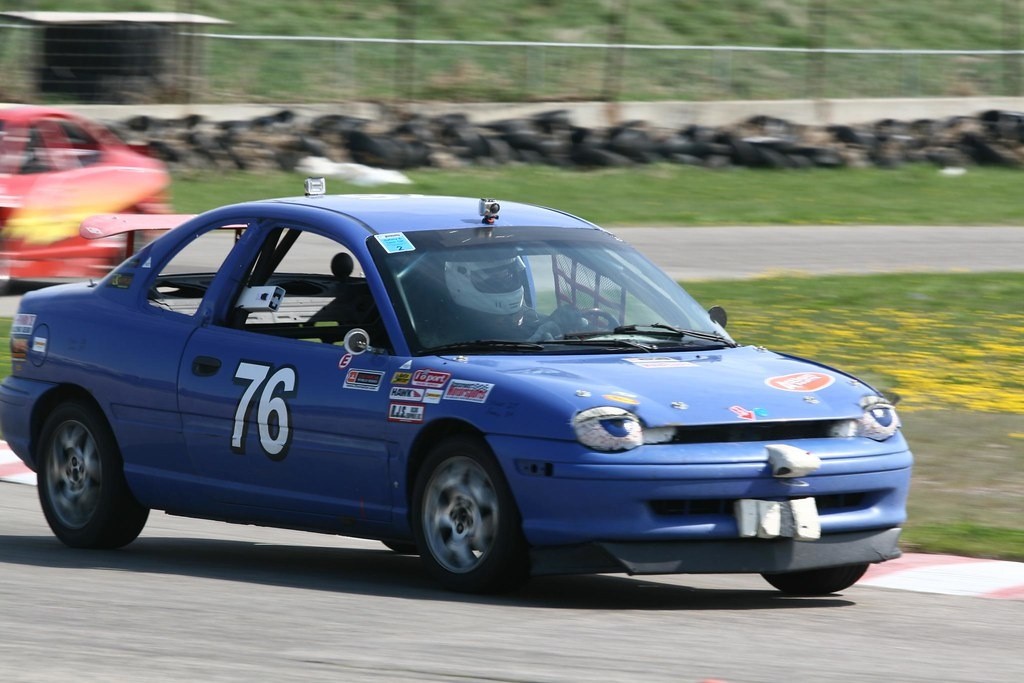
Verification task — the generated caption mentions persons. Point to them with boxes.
[444,245,588,343]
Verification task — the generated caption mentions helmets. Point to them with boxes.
[444,256,526,315]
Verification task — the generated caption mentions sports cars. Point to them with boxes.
[0,110,179,288]
[0,195,914,595]
